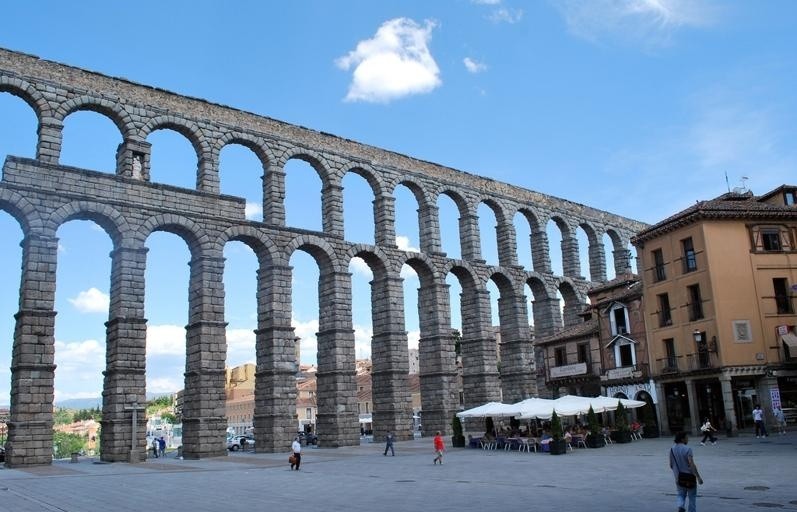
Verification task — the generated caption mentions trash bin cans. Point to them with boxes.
[71,453,78,463]
[301,437,307,447]
[178,446,183,457]
[244,441,249,451]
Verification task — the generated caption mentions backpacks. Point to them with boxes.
[701,425,709,432]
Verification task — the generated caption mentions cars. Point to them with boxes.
[297,430,317,447]
[227,435,255,451]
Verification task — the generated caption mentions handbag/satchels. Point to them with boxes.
[678,471,696,491]
[288,455,296,464]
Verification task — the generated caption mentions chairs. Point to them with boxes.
[479,425,644,453]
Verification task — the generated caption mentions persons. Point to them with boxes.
[752,404,765,439]
[774,406,787,434]
[700,417,717,445]
[382,430,395,456]
[669,431,703,512]
[484,420,641,452]
[290,436,300,471]
[433,431,445,465]
[152,436,165,458]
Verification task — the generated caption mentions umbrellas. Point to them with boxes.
[456,394,647,437]
